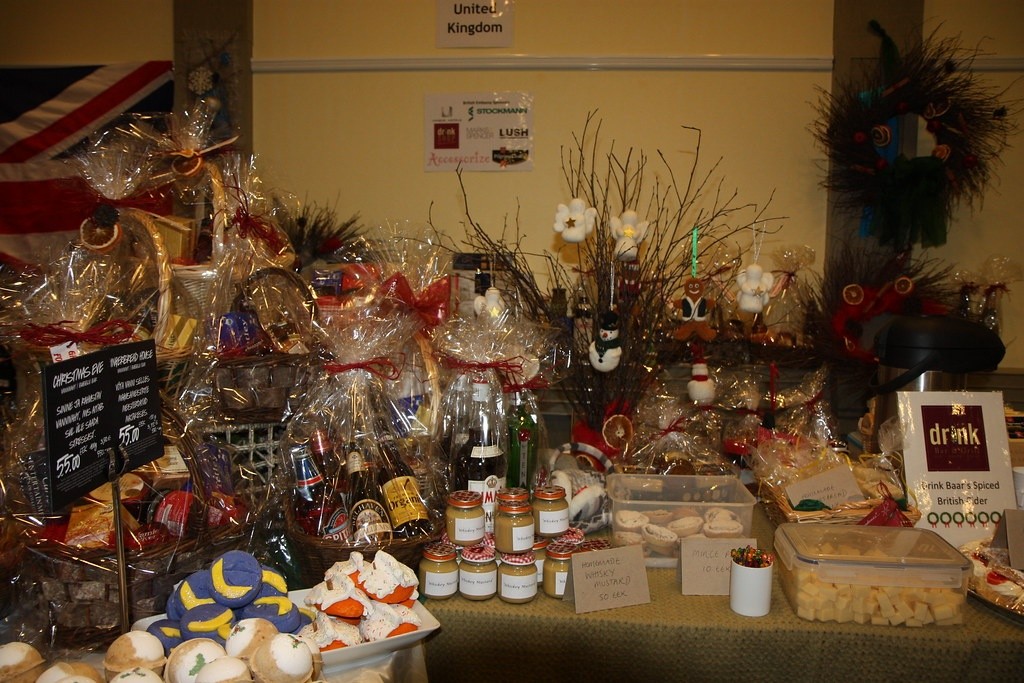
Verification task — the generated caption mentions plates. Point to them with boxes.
[288,589,441,673]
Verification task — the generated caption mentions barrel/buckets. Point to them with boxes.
[877,363,951,445]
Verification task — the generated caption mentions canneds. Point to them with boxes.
[420,485,615,603]
[75,475,206,551]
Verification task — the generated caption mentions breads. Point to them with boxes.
[614,507,746,559]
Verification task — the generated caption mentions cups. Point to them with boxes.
[730,558,773,616]
[1013,467,1024,509]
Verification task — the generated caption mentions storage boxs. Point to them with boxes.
[606,473,757,568]
[773,523,974,628]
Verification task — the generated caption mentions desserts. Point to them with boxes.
[0,551,418,683]
[958,546,1024,615]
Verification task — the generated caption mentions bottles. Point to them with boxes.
[578,280,593,310]
[294,389,534,543]
